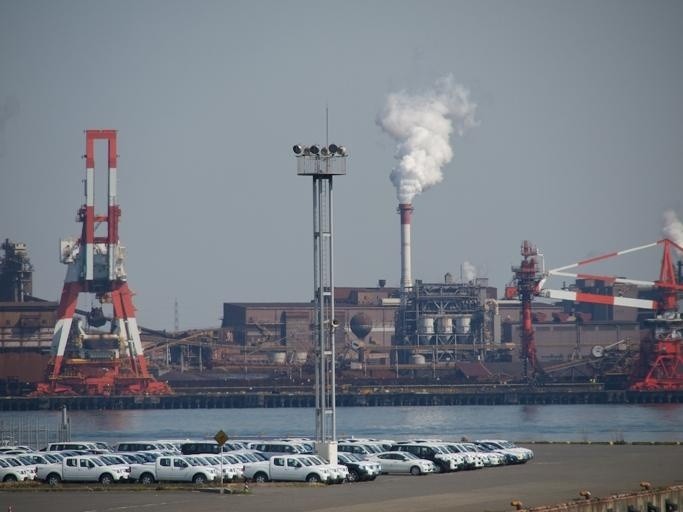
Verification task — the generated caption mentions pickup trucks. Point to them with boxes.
[0,435,535,487]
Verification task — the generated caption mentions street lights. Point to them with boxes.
[291,104,348,469]
